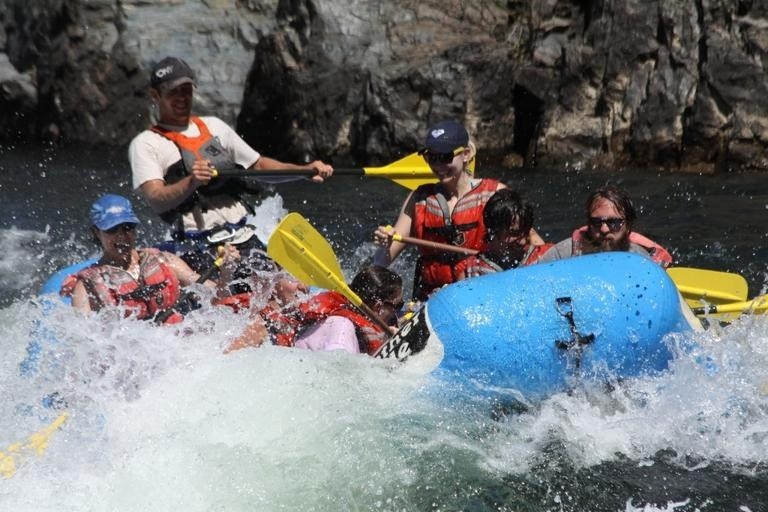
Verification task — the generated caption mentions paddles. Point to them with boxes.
[267,212,394,338]
[212,146,476,192]
[0,246,236,481]
[384,226,768,324]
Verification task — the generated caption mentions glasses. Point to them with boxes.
[97,223,137,233]
[589,218,626,232]
[383,300,405,312]
[425,147,464,164]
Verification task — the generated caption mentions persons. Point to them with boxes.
[58,193,218,327]
[453,188,556,281]
[373,120,545,302]
[290,264,403,354]
[226,272,311,353]
[128,56,334,296]
[529,186,673,273]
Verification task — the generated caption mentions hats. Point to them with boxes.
[418,123,468,155]
[89,194,140,230]
[151,56,197,94]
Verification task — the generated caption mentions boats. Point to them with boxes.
[21,251,720,419]
